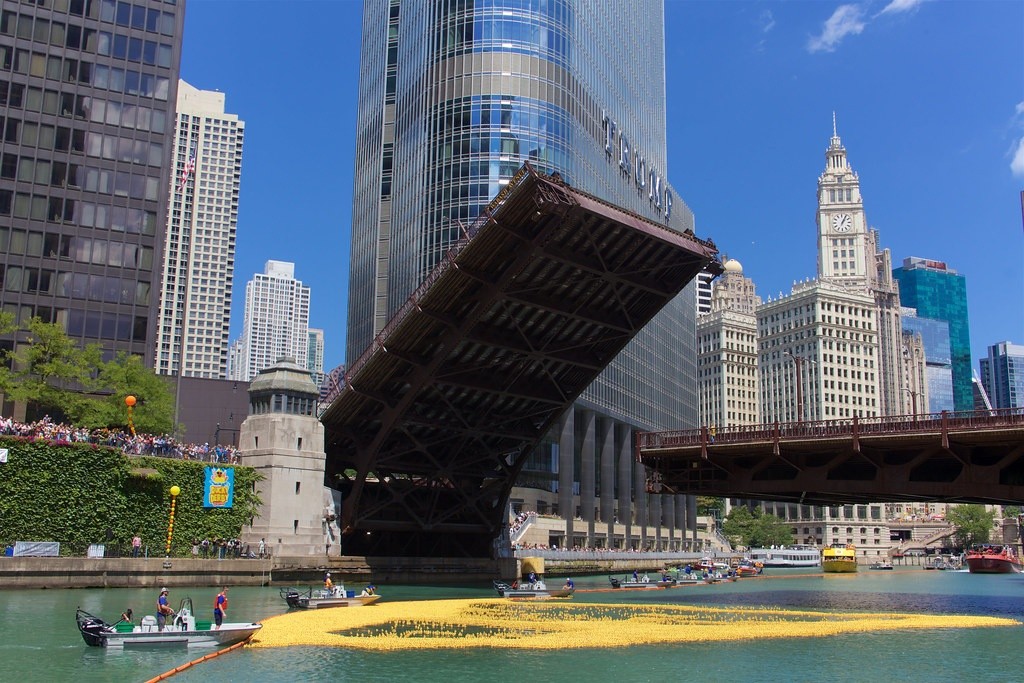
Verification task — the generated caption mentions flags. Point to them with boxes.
[176,149,195,191]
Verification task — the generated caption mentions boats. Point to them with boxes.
[280,582,383,609]
[869,559,893,570]
[923,553,962,570]
[608,571,674,589]
[493,579,576,598]
[745,544,821,567]
[965,543,1024,573]
[673,556,763,586]
[75,595,263,649]
[820,543,858,573]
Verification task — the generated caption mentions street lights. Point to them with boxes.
[783,352,817,426]
[901,387,925,422]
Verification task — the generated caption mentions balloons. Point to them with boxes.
[125,396,136,406]
[165,485,181,553]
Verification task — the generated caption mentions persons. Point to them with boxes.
[361,585,377,596]
[325,572,335,594]
[632,570,638,580]
[192,538,200,559]
[121,608,133,624]
[563,577,574,591]
[969,545,1014,560]
[889,513,945,524]
[212,536,218,558]
[258,538,266,560]
[528,571,538,584]
[202,538,210,559]
[762,544,798,551]
[156,587,173,631]
[219,537,256,559]
[512,580,519,589]
[132,535,142,558]
[710,423,716,444]
[213,586,228,630]
[510,511,690,553]
[661,560,763,581]
[0,414,239,465]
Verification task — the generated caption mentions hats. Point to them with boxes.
[161,587,169,592]
